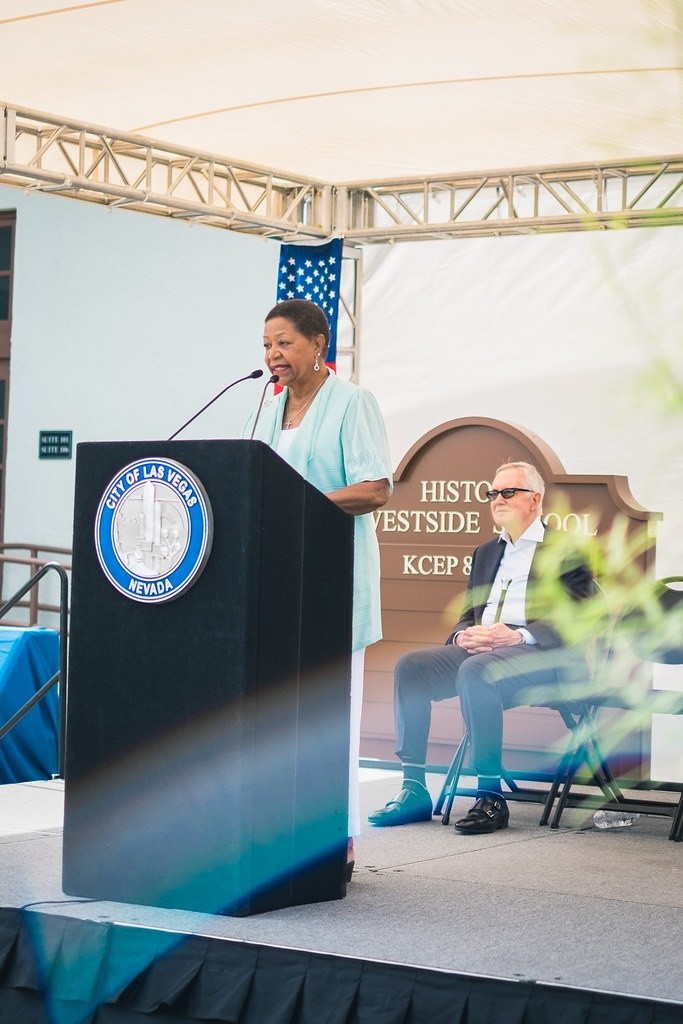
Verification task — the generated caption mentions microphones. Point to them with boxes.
[250,375,280,439]
[168,370,264,440]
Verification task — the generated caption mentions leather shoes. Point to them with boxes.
[368,790,433,826]
[454,798,509,834]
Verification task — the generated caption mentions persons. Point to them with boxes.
[238,300,394,883]
[367,461,593,834]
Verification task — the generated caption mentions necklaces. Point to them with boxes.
[284,374,329,430]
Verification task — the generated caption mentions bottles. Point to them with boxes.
[593,810,641,829]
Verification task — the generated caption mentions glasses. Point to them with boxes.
[484,488,534,501]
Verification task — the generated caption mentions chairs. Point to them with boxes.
[432,582,622,830]
[550,575,683,840]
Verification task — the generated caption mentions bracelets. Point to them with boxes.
[519,636,526,644]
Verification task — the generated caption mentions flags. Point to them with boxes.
[273,233,345,396]
